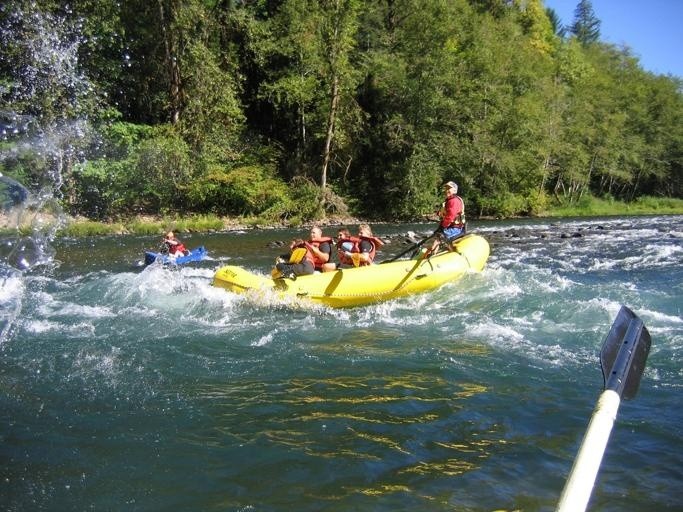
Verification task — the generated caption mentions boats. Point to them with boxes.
[214,234,490,307]
[145,246,207,265]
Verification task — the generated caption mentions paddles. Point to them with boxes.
[289,246,306,264]
[379,233,437,264]
[553,305,652,512]
[339,250,360,268]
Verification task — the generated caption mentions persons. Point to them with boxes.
[277,239,315,275]
[335,229,350,263]
[340,224,384,268]
[306,226,333,271]
[422,181,466,259]
[166,231,189,258]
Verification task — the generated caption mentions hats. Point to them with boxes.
[443,181,458,193]
